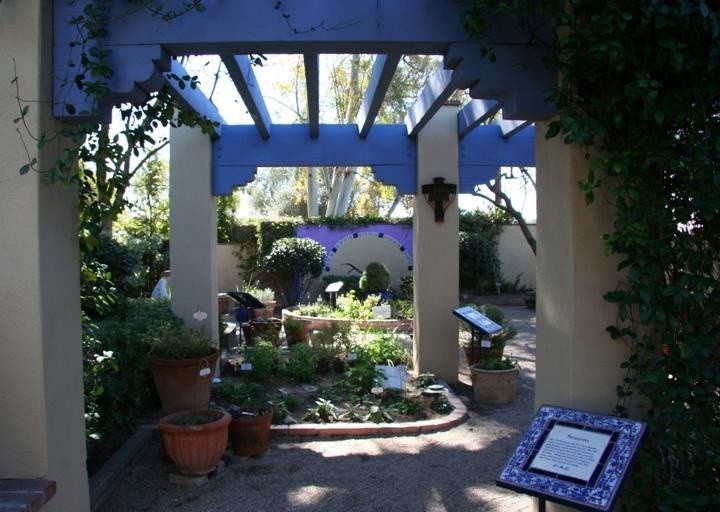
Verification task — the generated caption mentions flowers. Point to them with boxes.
[135,301,222,356]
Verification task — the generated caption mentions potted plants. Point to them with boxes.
[223,271,260,321]
[213,380,275,459]
[466,317,522,407]
[158,407,233,479]
[461,301,506,369]
[239,284,279,319]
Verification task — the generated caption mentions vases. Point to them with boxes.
[146,347,219,418]
[284,318,310,347]
[241,317,282,347]
